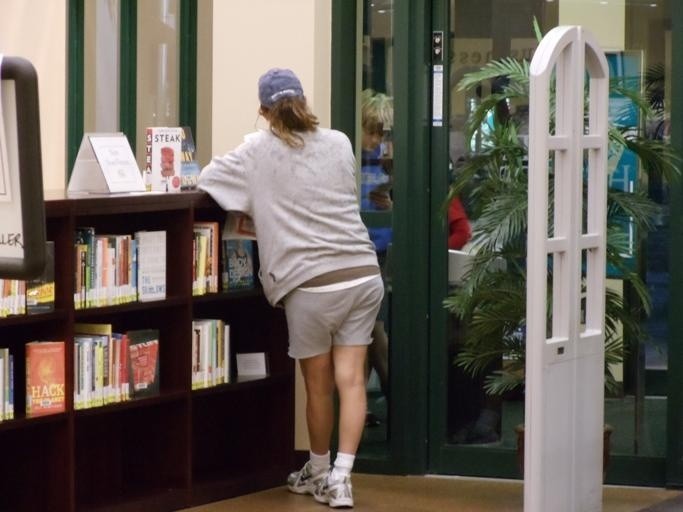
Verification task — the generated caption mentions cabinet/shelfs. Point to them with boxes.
[0,189,297,512]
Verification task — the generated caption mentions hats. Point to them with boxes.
[258,67,304,110]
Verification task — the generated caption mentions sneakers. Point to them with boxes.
[287,459,354,508]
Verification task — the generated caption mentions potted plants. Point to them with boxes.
[436,11,682,487]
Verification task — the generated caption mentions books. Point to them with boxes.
[1,241,55,318]
[191,319,230,390]
[193,222,253,295]
[74,323,159,410]
[73,227,167,309]
[0,341,64,422]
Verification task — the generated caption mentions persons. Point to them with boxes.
[353,85,392,428]
[195,65,383,508]
